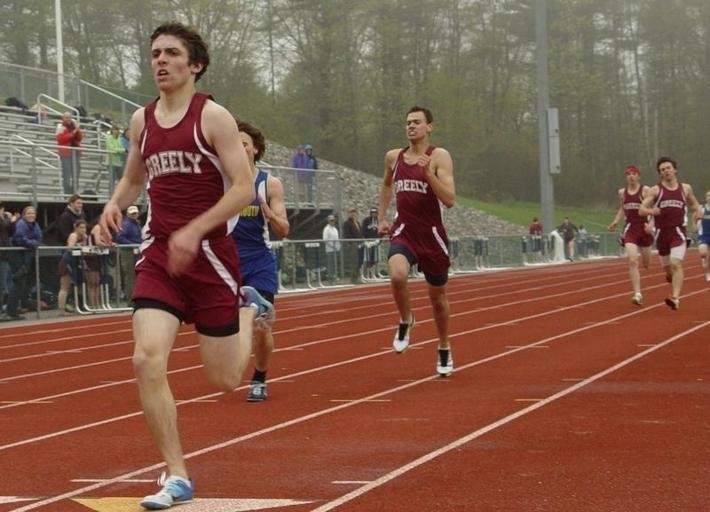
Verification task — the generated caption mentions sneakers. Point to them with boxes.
[393,316,415,353]
[139,476,196,507]
[246,376,268,402]
[239,287,273,316]
[630,291,642,307]
[665,295,681,311]
[437,342,456,378]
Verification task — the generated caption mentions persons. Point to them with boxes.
[60,196,85,244]
[226,121,289,401]
[558,217,578,262]
[58,221,86,317]
[87,219,113,312]
[695,191,707,281]
[103,123,137,198]
[12,206,41,314]
[374,105,456,376]
[57,112,82,195]
[115,205,142,302]
[638,158,703,309]
[529,218,541,234]
[363,207,382,281]
[293,141,319,201]
[609,165,654,306]
[325,216,341,281]
[1,205,26,320]
[100,23,276,507]
[341,207,366,282]
[578,226,588,253]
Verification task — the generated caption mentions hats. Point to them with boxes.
[127,206,140,215]
[305,144,312,150]
